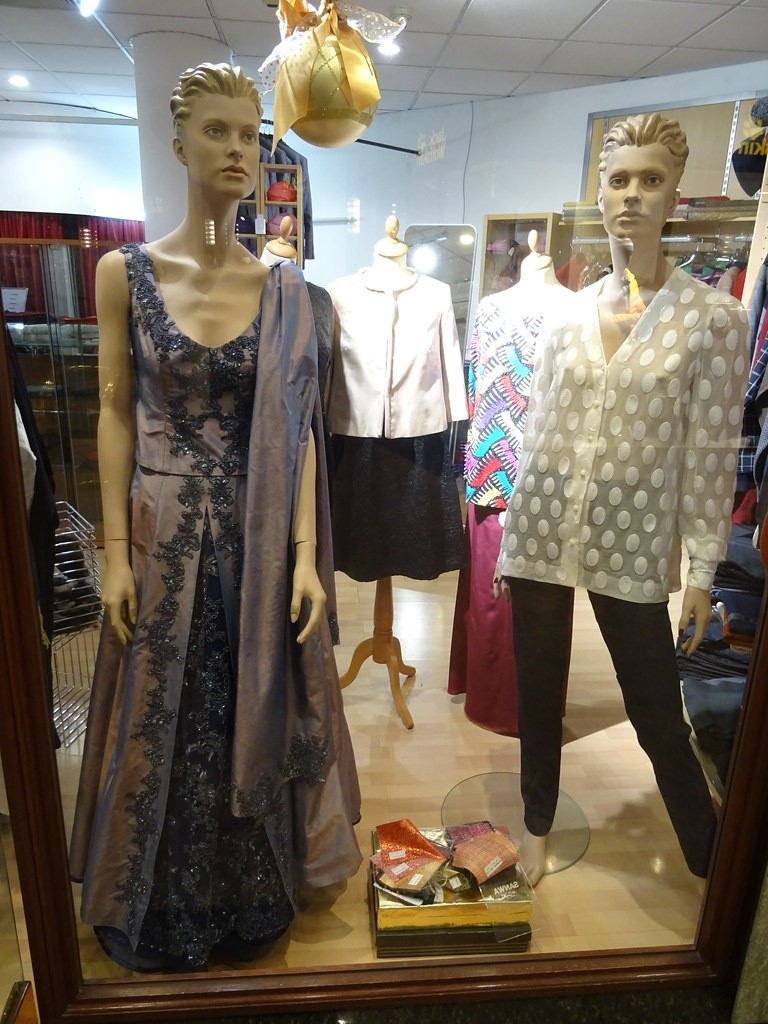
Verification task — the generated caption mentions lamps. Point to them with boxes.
[258,2,407,158]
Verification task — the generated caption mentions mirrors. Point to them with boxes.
[402,225,479,468]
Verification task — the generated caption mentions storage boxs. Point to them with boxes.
[365,822,537,958]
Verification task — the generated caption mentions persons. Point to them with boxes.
[0,313,63,748]
[251,236,580,739]
[95,63,327,972]
[497,113,751,892]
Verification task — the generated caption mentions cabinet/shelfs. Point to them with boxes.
[467,213,561,366]
